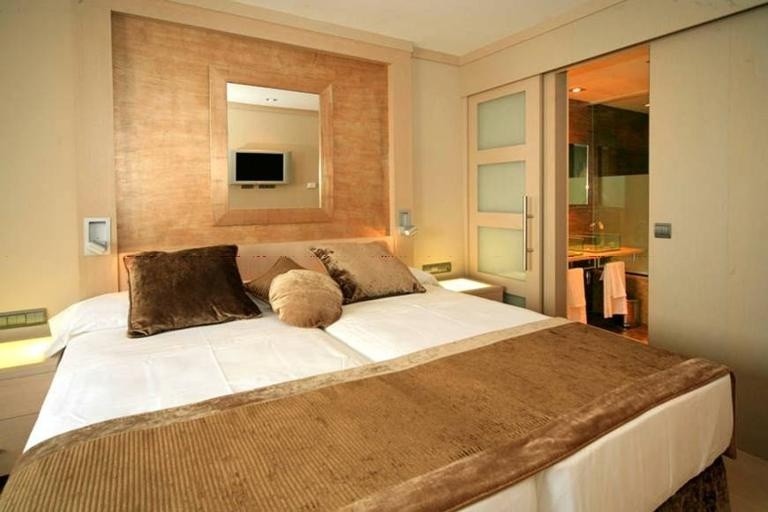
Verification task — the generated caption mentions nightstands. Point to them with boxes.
[0,323,64,476]
[438,277,505,302]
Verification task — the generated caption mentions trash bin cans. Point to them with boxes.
[624,300,641,330]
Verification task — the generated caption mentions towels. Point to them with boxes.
[568,142,590,206]
[603,261,628,318]
[567,268,587,324]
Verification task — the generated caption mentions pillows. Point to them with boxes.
[235,244,330,283]
[306,243,426,303]
[269,269,341,329]
[46,291,131,355]
[122,240,261,339]
[244,258,305,302]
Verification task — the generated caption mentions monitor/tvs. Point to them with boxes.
[226,148,291,186]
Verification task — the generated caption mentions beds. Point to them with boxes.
[0,284,737,512]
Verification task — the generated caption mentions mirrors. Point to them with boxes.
[208,64,335,225]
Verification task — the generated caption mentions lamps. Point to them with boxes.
[84,217,111,257]
[398,208,419,240]
